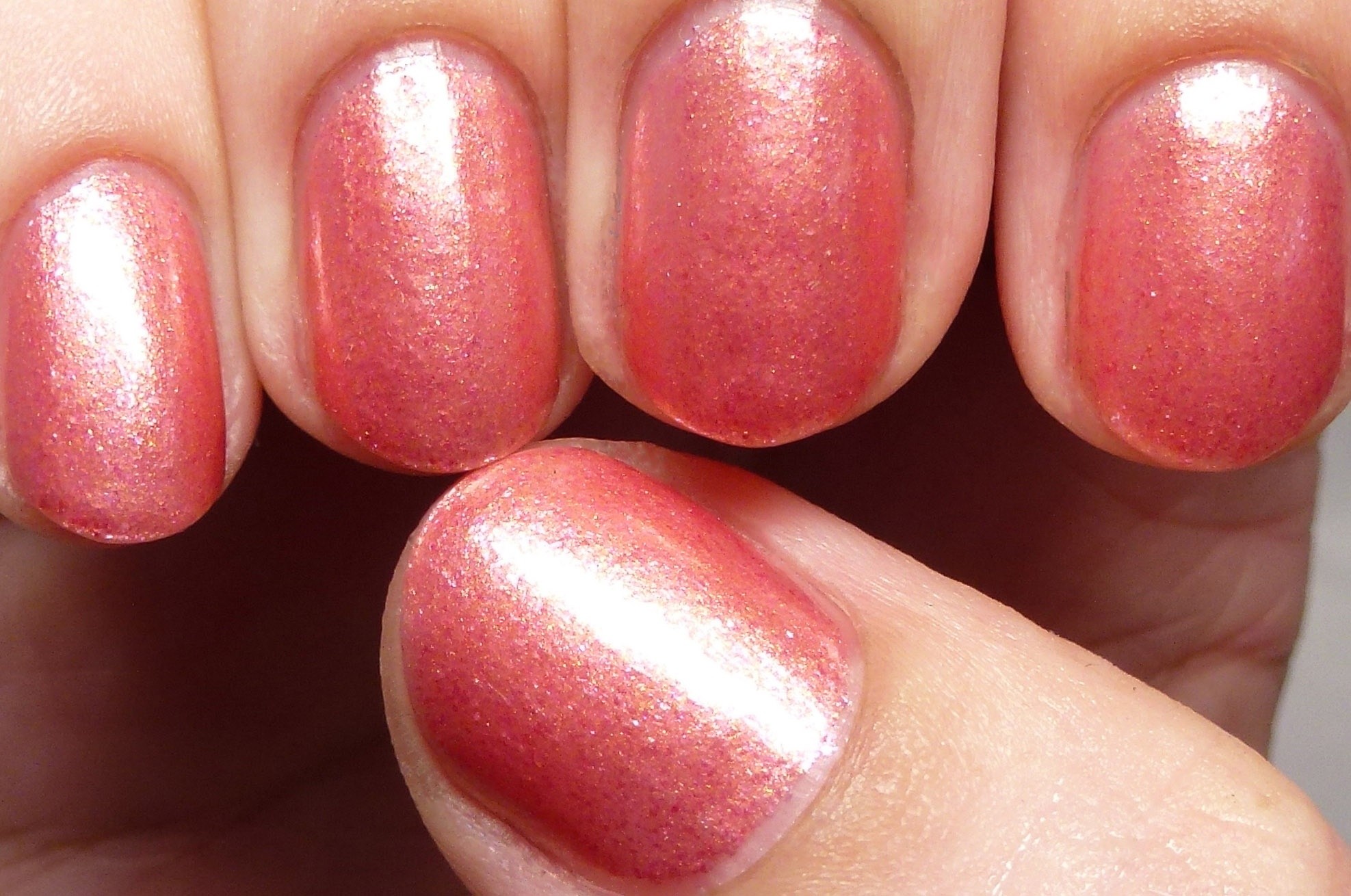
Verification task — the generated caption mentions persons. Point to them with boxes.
[1,0,1351,895]
